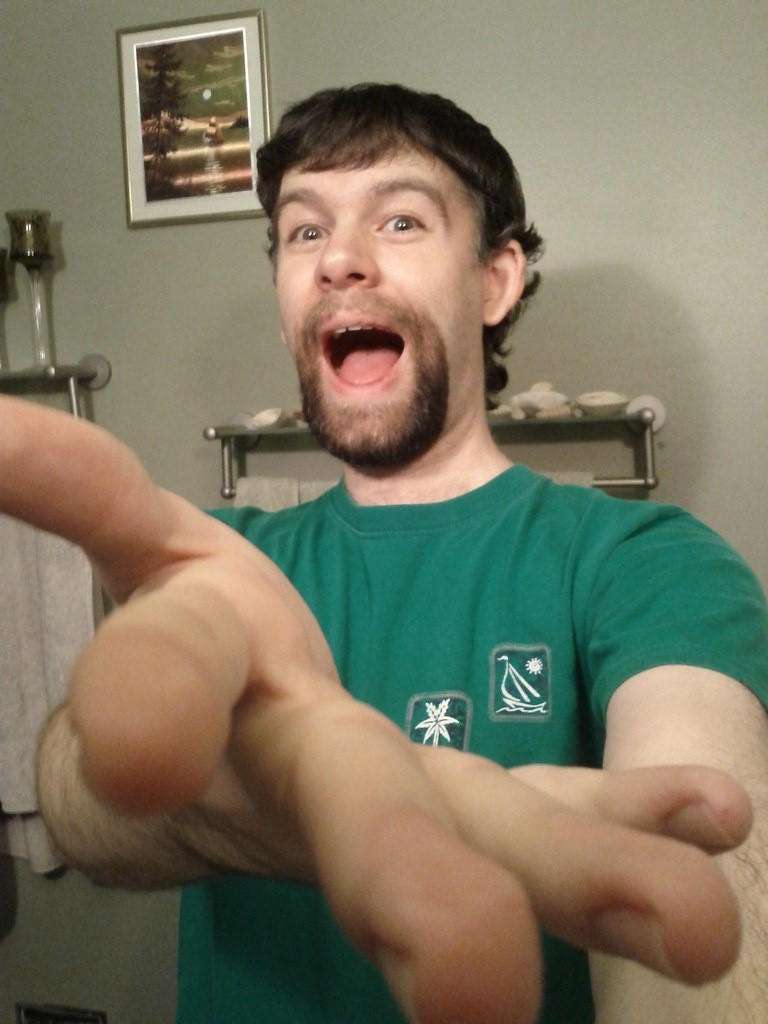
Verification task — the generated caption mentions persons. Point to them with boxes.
[0,81,768,1024]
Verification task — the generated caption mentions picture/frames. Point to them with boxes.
[114,7,275,231]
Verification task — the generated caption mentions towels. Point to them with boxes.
[0,495,148,877]
